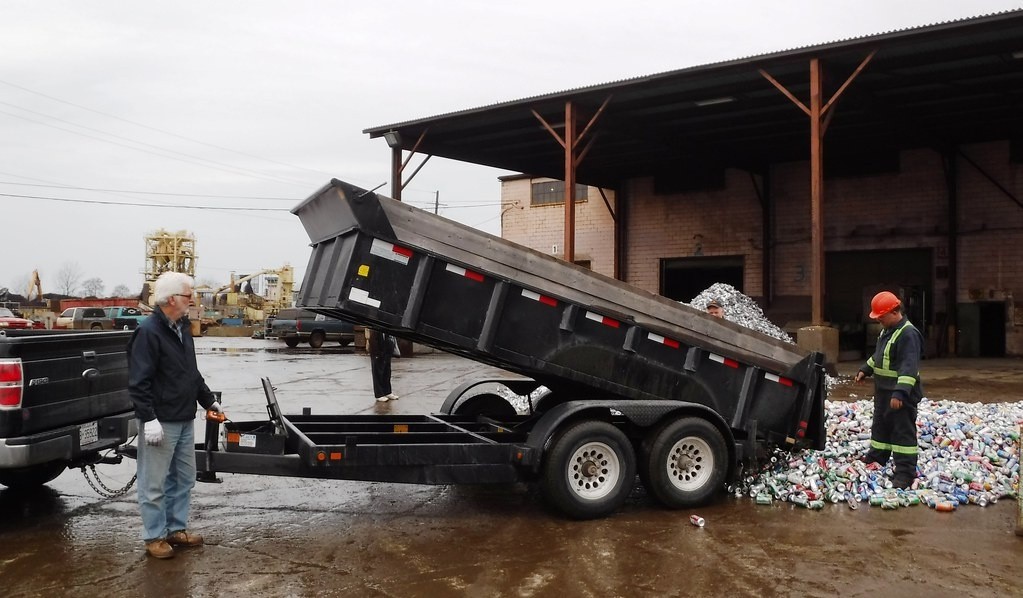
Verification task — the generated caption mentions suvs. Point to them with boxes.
[263,306,374,348]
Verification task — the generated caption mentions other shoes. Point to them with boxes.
[386,394,399,400]
[376,396,389,401]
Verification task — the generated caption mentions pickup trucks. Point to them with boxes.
[0,329,137,487]
[82,306,154,331]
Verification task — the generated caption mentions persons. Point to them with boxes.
[125,272,224,559]
[369,323,399,402]
[706,300,726,321]
[851,290,924,490]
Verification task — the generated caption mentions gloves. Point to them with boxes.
[208,402,224,416]
[145,418,164,446]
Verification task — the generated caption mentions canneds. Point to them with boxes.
[690,515,705,526]
[724,399,1023,511]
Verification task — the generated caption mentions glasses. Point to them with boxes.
[172,293,191,299]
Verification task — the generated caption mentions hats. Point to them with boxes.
[706,299,723,308]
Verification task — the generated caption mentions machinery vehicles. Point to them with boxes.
[27,266,52,309]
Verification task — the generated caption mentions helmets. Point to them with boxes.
[869,291,901,319]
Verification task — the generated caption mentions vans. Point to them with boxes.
[56,306,106,330]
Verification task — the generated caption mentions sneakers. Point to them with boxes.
[167,531,204,546]
[145,540,174,558]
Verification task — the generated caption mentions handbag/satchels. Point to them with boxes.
[390,336,400,356]
